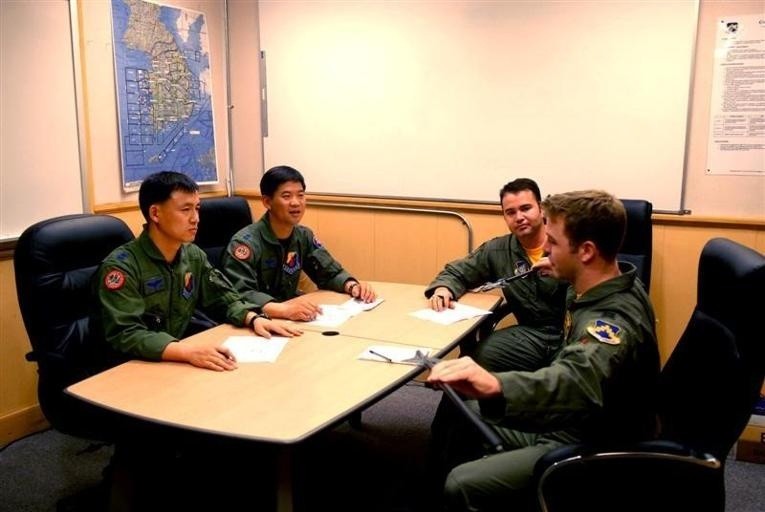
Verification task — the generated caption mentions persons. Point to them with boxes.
[90,170,303,374]
[426,189,663,510]
[219,166,377,322]
[424,177,572,445]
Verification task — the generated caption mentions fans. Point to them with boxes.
[258,0,702,215]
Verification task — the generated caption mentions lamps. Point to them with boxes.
[193,197,251,324]
[535,239,765,512]
[620,199,652,295]
[15,213,211,445]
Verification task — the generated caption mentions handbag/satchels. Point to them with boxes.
[348,283,360,294]
[249,313,270,329]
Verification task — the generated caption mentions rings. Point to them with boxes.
[437,294,458,302]
[220,352,235,362]
[369,350,392,363]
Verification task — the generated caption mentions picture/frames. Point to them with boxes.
[65,280,502,512]
